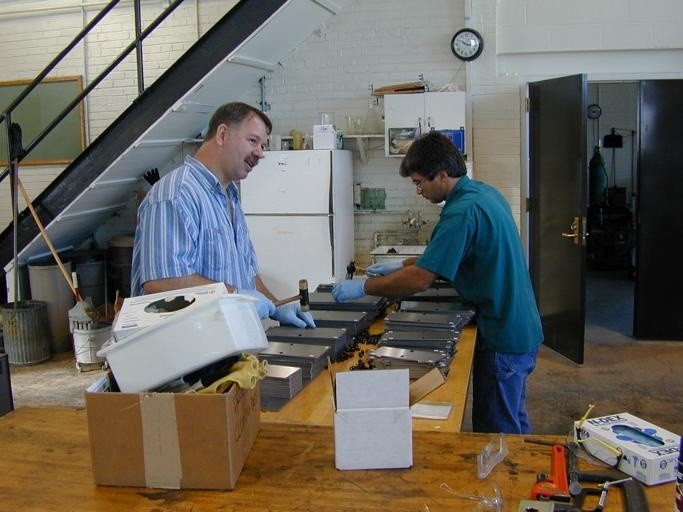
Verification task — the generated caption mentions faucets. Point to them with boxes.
[406,209,423,232]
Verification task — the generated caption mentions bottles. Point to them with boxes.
[336,128,343,150]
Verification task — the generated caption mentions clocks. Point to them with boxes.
[450,28,484,61]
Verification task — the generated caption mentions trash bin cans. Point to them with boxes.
[586,234,613,271]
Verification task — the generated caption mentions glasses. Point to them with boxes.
[411,173,428,191]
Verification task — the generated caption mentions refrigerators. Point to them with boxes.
[239,149,355,301]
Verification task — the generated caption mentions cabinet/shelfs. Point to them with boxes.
[384,91,466,158]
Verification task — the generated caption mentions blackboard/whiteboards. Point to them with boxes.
[1,75,86,165]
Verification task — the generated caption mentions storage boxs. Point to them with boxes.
[96,293,271,394]
[84,354,260,491]
[326,355,446,470]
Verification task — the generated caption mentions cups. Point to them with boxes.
[292,131,303,150]
[268,134,282,151]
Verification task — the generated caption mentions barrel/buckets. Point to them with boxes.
[73,322,112,373]
[0,301,52,365]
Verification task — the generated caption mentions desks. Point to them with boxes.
[263,303,477,432]
[0,405,676,512]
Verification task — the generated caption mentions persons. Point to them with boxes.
[129,101,319,329]
[330,129,545,435]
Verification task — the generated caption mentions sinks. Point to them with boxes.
[370,245,426,257]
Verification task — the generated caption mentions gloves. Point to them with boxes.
[331,278,370,305]
[237,289,277,320]
[270,299,317,329]
[365,260,405,278]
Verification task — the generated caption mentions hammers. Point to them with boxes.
[276,280,310,312]
[525,437,578,451]
[346,259,381,280]
